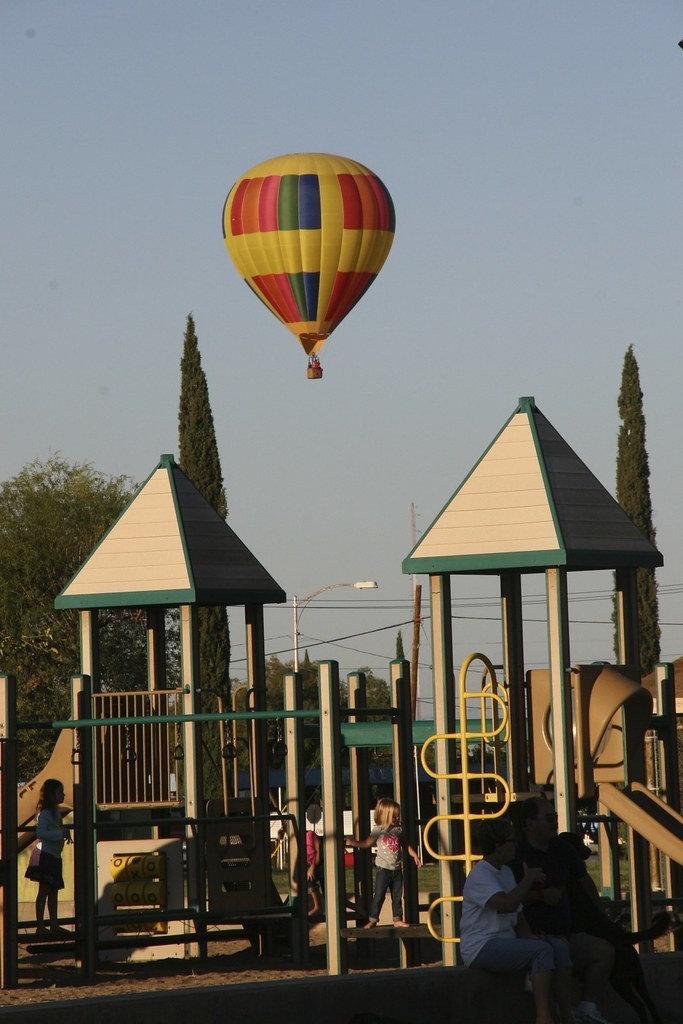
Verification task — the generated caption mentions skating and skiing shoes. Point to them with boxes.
[572,1007,607,1024]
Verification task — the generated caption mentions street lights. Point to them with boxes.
[293,581,379,673]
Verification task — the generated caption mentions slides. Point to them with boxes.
[594,780,683,866]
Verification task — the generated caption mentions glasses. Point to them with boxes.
[532,814,558,823]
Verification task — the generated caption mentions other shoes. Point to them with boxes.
[35,926,70,933]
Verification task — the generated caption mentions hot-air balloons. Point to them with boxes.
[222,152,397,356]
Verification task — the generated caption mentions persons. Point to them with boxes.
[461,796,616,1024]
[305,830,325,917]
[24,779,74,935]
[345,799,423,929]
